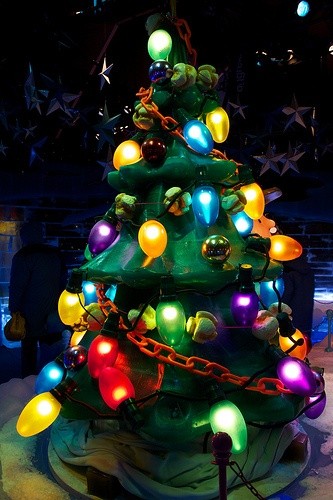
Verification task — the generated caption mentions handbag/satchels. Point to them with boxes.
[4,311,26,341]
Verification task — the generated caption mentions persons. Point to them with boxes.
[9,223,68,379]
[279,252,314,349]
